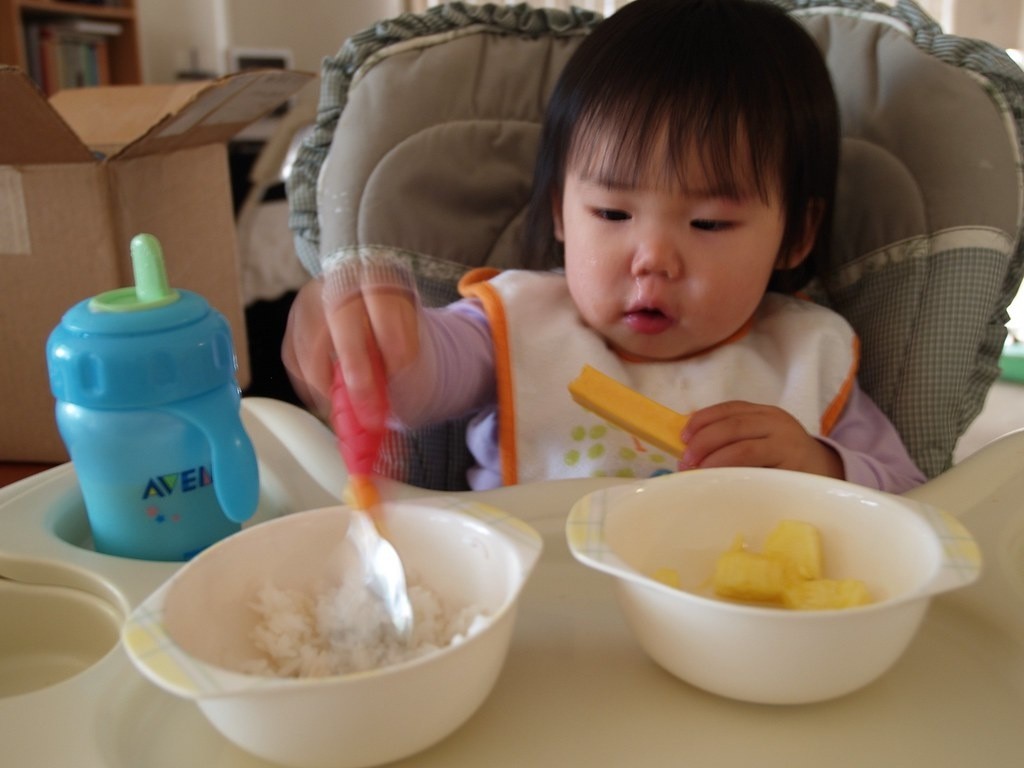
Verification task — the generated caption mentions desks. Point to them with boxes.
[0,396,1023,768]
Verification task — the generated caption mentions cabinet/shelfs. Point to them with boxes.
[0,0,142,93]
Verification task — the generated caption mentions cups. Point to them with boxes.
[0,556,130,768]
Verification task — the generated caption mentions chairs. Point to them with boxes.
[236,111,315,311]
[283,0,1024,491]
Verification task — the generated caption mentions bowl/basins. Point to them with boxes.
[563,467,983,710]
[121,492,544,768]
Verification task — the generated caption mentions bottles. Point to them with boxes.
[47,232,263,559]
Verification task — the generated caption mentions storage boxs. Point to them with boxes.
[0,65,318,464]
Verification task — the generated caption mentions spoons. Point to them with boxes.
[331,345,414,642]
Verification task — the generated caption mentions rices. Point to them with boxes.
[222,579,493,678]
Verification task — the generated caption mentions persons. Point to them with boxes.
[282,0,924,491]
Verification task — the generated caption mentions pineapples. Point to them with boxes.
[643,518,872,613]
